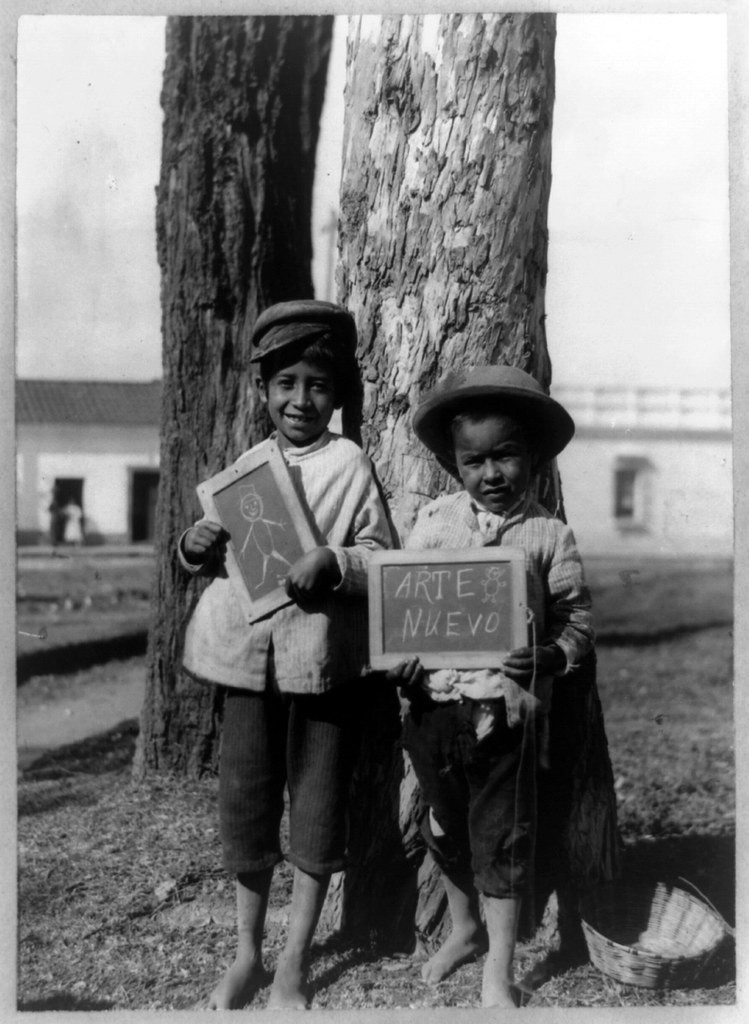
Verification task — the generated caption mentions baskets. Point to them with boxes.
[577,878,725,989]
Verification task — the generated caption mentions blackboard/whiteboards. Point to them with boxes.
[195,438,319,624]
[368,550,529,670]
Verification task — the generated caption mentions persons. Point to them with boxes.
[46,496,86,555]
[385,367,593,1009]
[178,300,396,1013]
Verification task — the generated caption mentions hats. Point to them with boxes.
[249,300,354,364]
[412,365,575,467]
